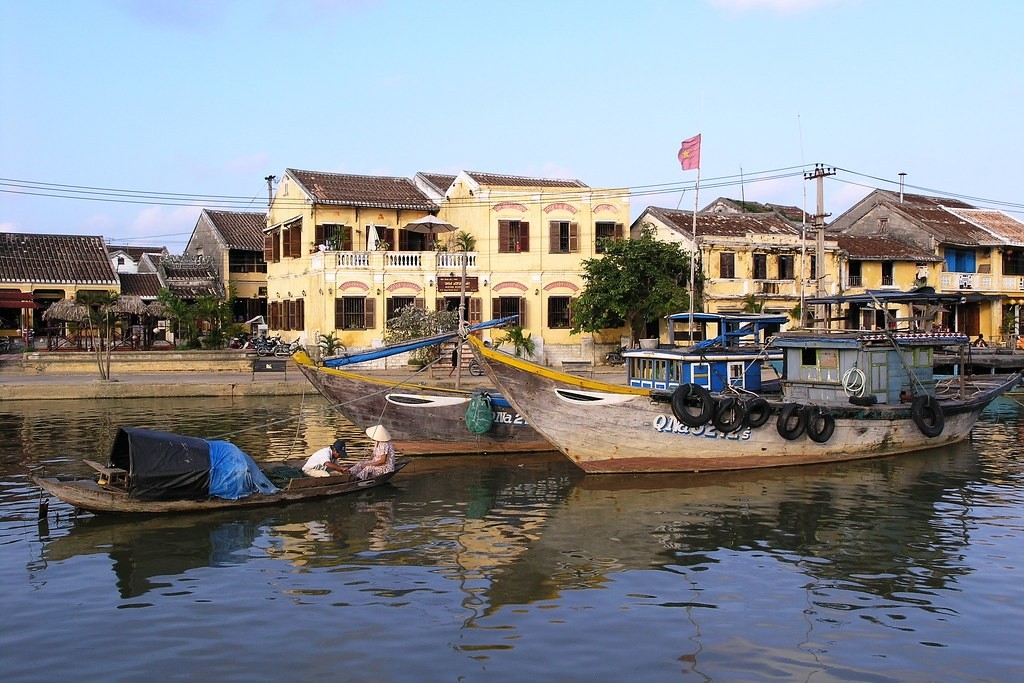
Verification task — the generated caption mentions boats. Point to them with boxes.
[291,314,562,455]
[32,426,412,512]
[465,312,1022,468]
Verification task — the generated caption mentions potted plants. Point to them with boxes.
[407,358,422,372]
[638,335,658,350]
[315,331,347,356]
[377,239,389,251]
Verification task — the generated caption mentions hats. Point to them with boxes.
[365,424,391,442]
[484,341,489,344]
[334,440,347,458]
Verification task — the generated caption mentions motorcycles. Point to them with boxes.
[0,336,20,354]
[251,333,305,358]
[469,357,484,377]
[605,345,628,367]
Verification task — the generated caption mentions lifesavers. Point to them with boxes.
[777,402,808,441]
[911,394,945,437]
[712,397,744,432]
[806,407,835,443]
[671,383,714,428]
[743,397,771,429]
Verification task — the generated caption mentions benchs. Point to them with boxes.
[85,460,128,489]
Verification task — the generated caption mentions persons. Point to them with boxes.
[448,342,463,378]
[972,334,988,348]
[302,441,349,478]
[349,425,395,480]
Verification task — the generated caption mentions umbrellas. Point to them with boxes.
[403,213,460,241]
[367,222,381,251]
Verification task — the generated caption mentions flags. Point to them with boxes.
[678,134,701,171]
